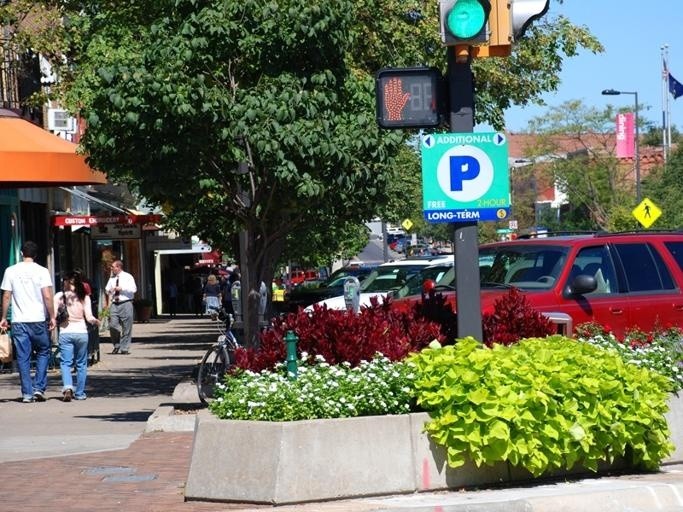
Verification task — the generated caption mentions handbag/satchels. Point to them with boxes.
[0,328,14,363]
[55,289,69,325]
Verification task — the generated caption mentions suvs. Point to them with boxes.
[378,227,682,344]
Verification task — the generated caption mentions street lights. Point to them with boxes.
[514,158,561,224]
[599,89,643,233]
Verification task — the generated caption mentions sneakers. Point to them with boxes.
[112,347,129,355]
[22,390,87,402]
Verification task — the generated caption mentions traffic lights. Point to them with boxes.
[487,0,550,47]
[375,65,441,128]
[439,0,492,49]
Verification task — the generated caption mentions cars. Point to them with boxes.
[283,235,551,348]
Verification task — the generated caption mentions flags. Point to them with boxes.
[664,59,683,99]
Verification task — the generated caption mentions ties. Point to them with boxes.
[115,277,119,302]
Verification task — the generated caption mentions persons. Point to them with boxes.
[73,268,92,298]
[104,260,138,354]
[167,270,240,321]
[0,241,55,403]
[51,272,101,402]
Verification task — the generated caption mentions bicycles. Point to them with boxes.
[196,303,268,408]
